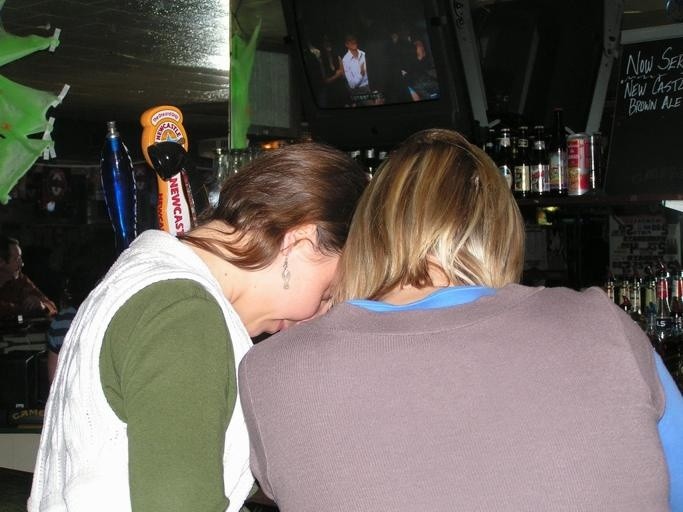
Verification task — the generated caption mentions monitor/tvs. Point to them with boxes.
[283,0,469,138]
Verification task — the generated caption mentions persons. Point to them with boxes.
[45,236,114,386]
[341,33,369,91]
[237,127,683,511]
[26,141,372,511]
[0,238,58,318]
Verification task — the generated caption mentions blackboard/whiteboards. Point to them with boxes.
[603,37,683,196]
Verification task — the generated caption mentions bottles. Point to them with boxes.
[484,128,497,160]
[514,126,530,198]
[567,132,594,195]
[549,108,566,194]
[498,129,514,196]
[670,270,683,375]
[229,145,240,178]
[607,278,614,303]
[630,276,647,332]
[530,126,549,198]
[652,272,679,380]
[365,147,374,181]
[204,146,227,207]
[644,275,656,349]
[618,280,631,306]
